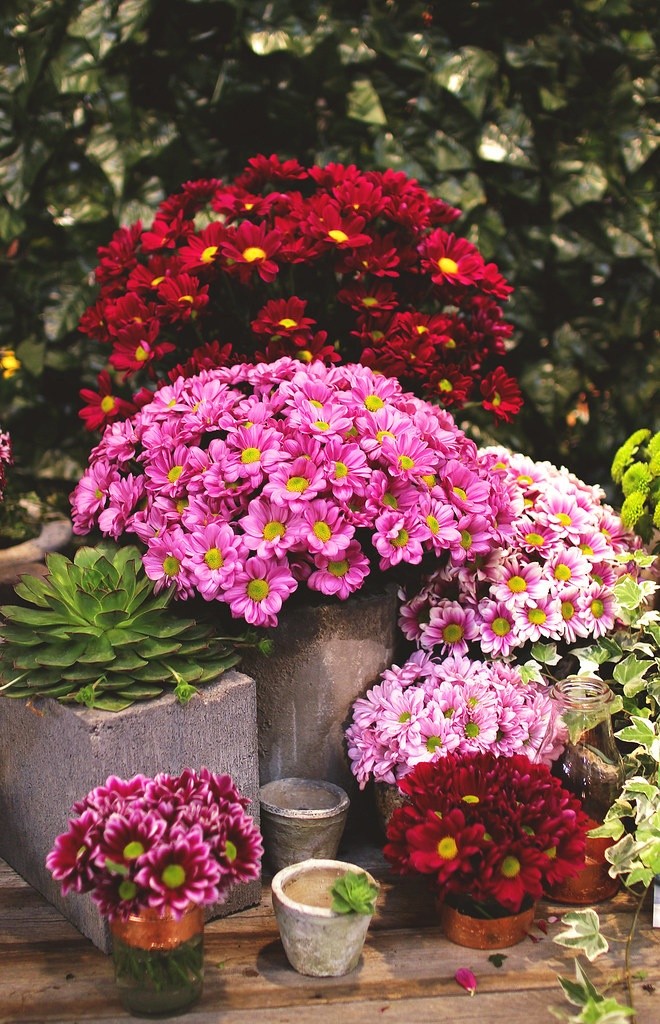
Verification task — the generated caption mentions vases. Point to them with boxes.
[112,926,203,1019]
[255,777,349,871]
[439,889,537,949]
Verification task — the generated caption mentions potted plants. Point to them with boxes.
[0,542,263,953]
[271,857,380,975]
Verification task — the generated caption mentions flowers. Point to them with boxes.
[48,157,650,926]
